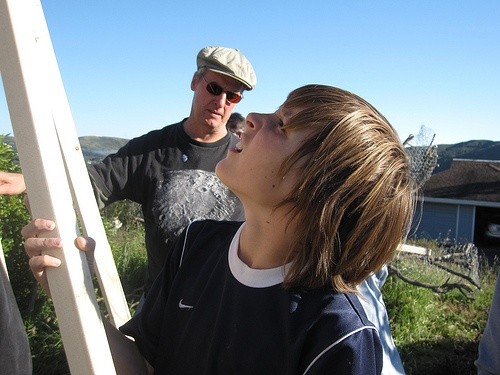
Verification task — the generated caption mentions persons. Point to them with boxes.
[19,83,422,375]
[0,44,258,319]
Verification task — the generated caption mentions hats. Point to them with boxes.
[197,46,257,90]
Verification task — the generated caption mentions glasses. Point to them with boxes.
[202,74,243,103]
